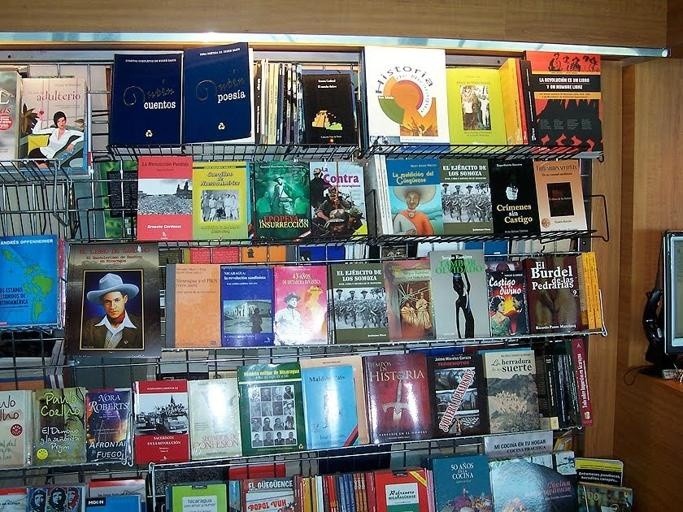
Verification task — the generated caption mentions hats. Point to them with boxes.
[393,184,435,204]
[283,291,300,303]
[204,192,236,200]
[254,308,260,313]
[86,273,139,305]
[442,182,489,194]
[553,52,596,64]
[272,174,284,182]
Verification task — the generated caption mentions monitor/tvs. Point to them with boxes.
[664,229,683,357]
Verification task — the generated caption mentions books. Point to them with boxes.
[64,238,634,512]
[0,234,90,512]
[1,42,604,180]
[94,151,589,246]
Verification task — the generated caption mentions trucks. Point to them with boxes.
[136,411,189,437]
